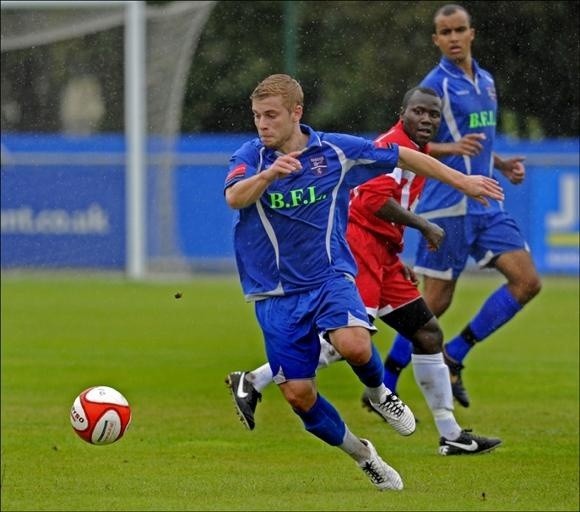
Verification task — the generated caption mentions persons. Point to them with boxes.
[358,4,542,427]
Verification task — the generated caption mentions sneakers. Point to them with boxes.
[438,429,503,455]
[225,371,261,431]
[361,384,418,436]
[442,342,469,408]
[355,438,404,492]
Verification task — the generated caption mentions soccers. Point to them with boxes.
[70,385,132,446]
